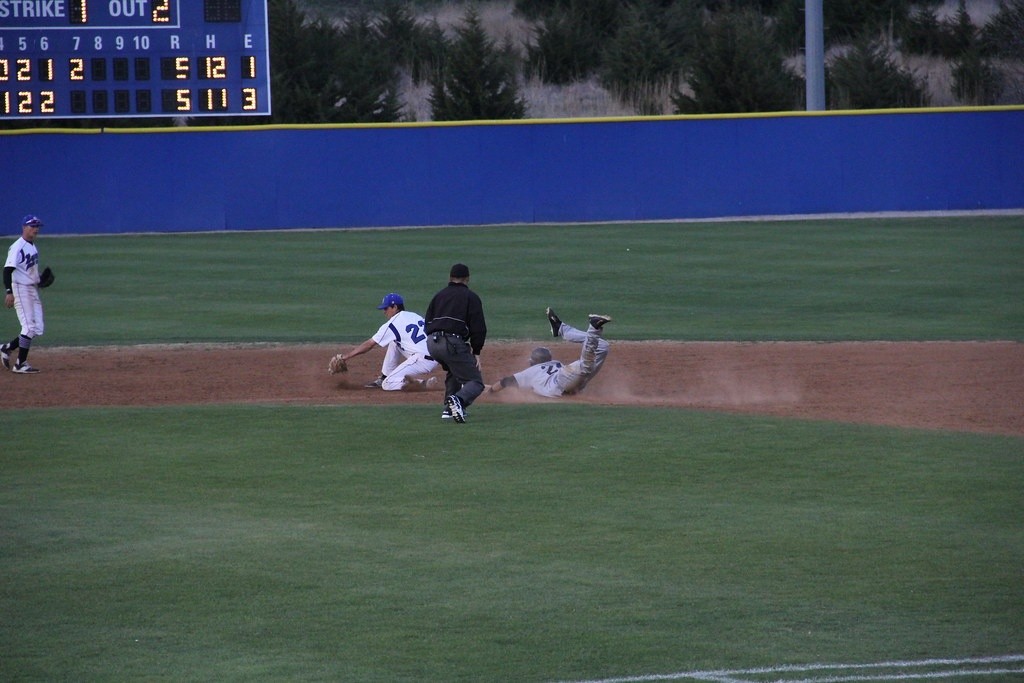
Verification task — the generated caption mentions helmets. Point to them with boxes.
[529,348,552,365]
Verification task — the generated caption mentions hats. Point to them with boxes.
[375,292,403,310]
[22,214,44,226]
[449,263,470,278]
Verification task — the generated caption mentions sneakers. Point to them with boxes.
[364,376,384,388]
[12,361,40,373]
[588,314,612,329]
[0,344,10,370]
[442,410,469,419]
[447,395,466,423]
[546,307,562,337]
[427,376,439,388]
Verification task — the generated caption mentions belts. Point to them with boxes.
[415,353,434,361]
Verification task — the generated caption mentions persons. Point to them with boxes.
[421,263,488,424]
[485,305,615,399]
[0,214,45,375]
[330,294,441,392]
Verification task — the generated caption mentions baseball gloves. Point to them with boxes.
[37,266,55,289]
[328,354,348,374]
